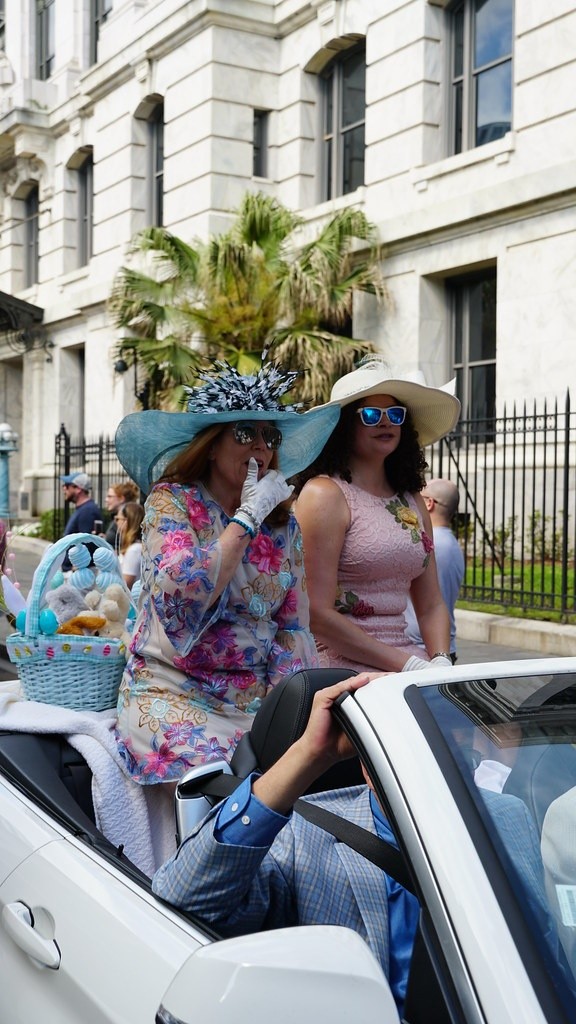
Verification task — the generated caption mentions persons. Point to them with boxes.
[403,478,465,665]
[92,482,138,547]
[151,672,559,1004]
[113,357,341,799]
[114,502,145,589]
[59,472,101,572]
[294,370,461,673]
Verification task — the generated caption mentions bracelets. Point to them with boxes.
[229,507,260,540]
[432,652,452,663]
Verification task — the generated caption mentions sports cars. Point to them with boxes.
[0,656,576,1024]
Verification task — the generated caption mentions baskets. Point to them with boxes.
[7,533,138,712]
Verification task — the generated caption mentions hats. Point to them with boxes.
[114,346,341,496]
[304,369,461,447]
[60,472,92,491]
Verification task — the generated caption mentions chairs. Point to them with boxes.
[175,665,367,843]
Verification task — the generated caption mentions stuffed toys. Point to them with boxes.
[16,546,132,661]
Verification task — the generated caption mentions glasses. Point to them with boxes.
[355,406,406,427]
[226,421,282,449]
[113,515,129,522]
[62,483,77,490]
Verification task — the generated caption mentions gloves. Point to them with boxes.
[228,457,295,539]
[401,654,452,672]
